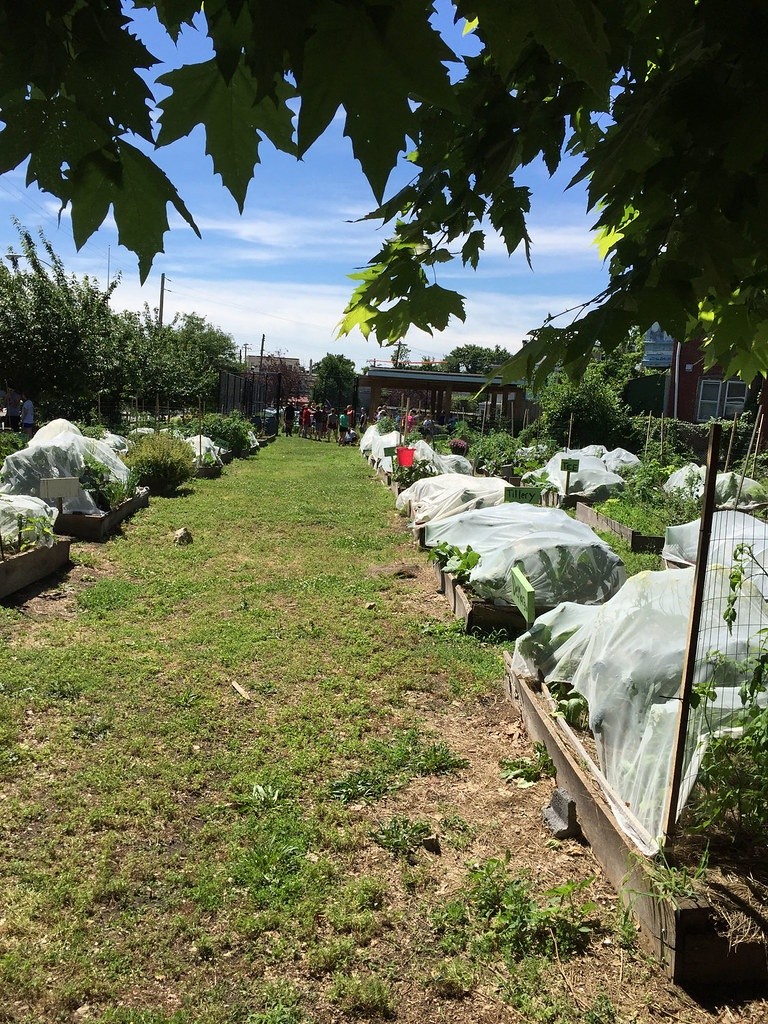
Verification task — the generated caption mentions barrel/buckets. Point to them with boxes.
[398,448,415,465]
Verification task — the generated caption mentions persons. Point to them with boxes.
[438,410,446,426]
[298,402,358,446]
[448,413,455,436]
[423,414,433,433]
[374,405,388,422]
[392,409,423,435]
[5,385,21,433]
[359,407,367,433]
[21,391,34,436]
[283,401,295,437]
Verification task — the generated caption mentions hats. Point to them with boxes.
[426,413,433,417]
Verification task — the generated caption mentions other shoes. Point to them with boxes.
[328,440,330,442]
[286,434,292,437]
[313,438,321,441]
